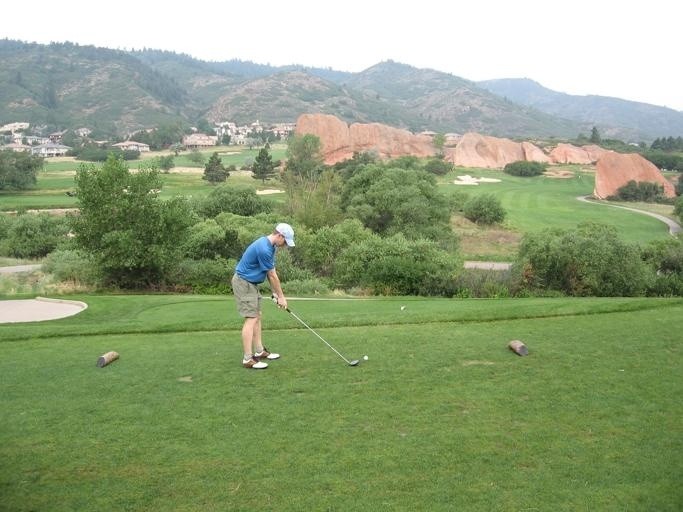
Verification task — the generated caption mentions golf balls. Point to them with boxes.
[363,355,368,360]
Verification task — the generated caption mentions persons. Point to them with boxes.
[231,223,295,369]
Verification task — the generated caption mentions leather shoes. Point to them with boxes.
[254,345,280,359]
[243,356,268,369]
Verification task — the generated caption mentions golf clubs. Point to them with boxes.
[272,296,359,366]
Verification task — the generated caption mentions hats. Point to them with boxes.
[275,223,296,247]
